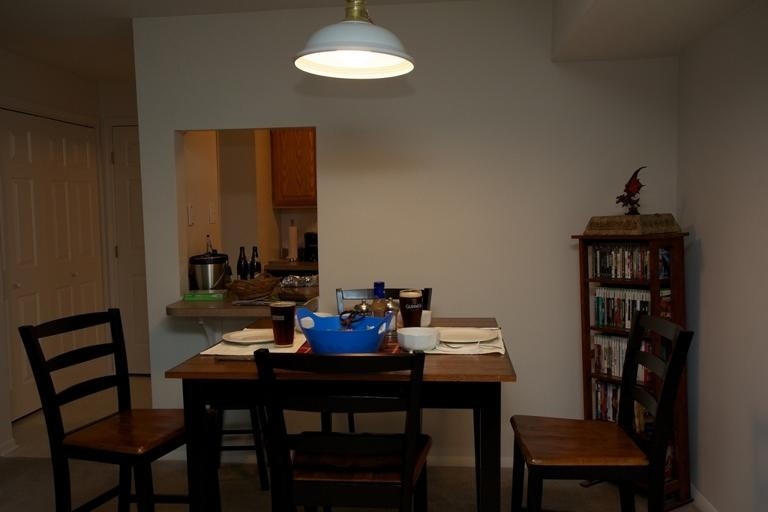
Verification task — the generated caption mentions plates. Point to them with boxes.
[438,327,500,344]
[221,329,276,345]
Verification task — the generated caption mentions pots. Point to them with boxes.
[188,252,233,290]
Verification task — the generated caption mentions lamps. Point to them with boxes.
[293,0,415,80]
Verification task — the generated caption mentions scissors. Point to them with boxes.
[339,309,366,329]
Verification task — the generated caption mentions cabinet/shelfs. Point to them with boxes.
[270,128,317,211]
[571,214,694,512]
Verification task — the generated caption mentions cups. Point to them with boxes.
[269,302,297,347]
[399,289,424,328]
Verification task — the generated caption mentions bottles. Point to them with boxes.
[372,280,398,337]
[250,246,262,280]
[236,246,249,280]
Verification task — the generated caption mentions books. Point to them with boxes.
[588,240,675,482]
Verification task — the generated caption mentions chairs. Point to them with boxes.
[510,312,694,512]
[248,348,432,512]
[18,308,223,512]
[321,288,433,511]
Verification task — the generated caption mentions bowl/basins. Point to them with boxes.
[295,313,334,332]
[395,326,440,352]
[294,306,393,353]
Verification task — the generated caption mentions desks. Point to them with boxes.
[167,280,318,318]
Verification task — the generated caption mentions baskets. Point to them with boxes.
[228,272,282,305]
